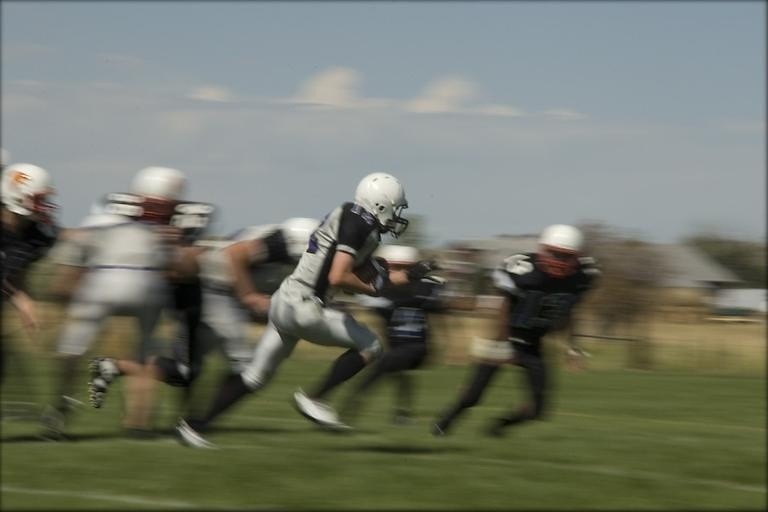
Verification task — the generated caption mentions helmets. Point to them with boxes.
[1,163,60,217]
[538,223,584,253]
[134,168,185,200]
[354,172,409,239]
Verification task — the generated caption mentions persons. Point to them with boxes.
[427,221,606,441]
[1,161,480,450]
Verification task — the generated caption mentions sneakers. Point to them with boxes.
[175,416,213,449]
[88,358,121,408]
[292,386,349,429]
